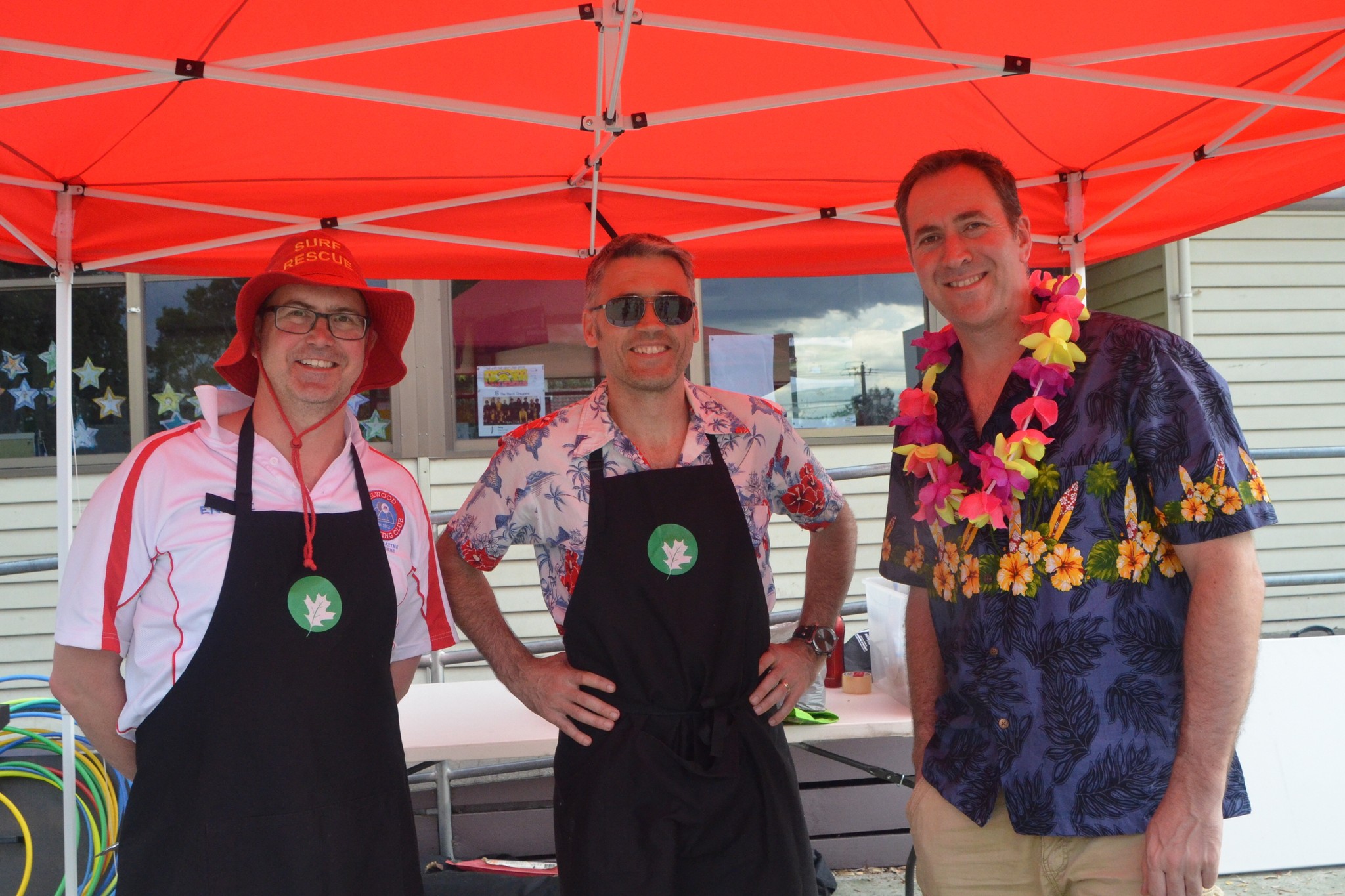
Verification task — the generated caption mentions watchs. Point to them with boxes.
[791,623,839,658]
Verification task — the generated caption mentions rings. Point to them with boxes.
[779,680,790,695]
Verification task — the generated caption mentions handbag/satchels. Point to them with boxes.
[783,707,839,725]
[770,621,826,711]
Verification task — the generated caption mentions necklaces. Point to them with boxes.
[890,268,1088,530]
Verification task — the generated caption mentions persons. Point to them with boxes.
[51,234,459,896]
[482,397,541,425]
[879,150,1282,896]
[436,232,858,896]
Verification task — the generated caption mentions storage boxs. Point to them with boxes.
[861,575,911,711]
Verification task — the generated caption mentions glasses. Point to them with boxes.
[259,306,371,340]
[591,295,696,326]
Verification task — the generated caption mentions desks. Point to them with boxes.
[396,679,916,896]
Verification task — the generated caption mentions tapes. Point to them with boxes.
[841,671,873,695]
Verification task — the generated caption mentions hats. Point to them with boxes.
[214,235,415,397]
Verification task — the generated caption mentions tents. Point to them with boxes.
[454,281,752,363]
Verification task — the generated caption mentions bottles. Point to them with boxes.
[824,614,844,688]
